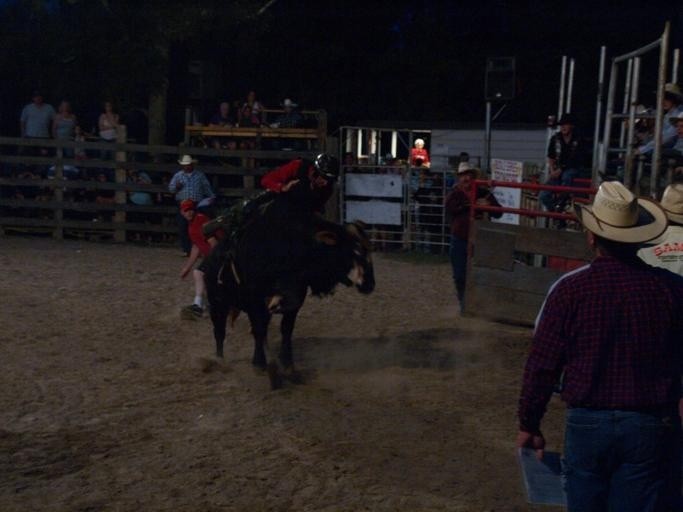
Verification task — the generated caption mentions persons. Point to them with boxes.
[222,152,339,265]
[205,89,312,191]
[171,155,228,318]
[518,181,683,512]
[23,90,160,246]
[408,158,447,257]
[446,162,505,312]
[536,83,681,275]
[344,153,403,175]
[410,139,431,168]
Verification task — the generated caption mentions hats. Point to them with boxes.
[454,161,482,179]
[279,98,298,109]
[178,198,195,211]
[176,155,198,166]
[571,179,668,248]
[656,182,683,225]
[650,82,682,98]
[556,113,583,126]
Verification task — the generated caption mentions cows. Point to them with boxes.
[201,208,376,371]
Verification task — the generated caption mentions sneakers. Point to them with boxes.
[179,303,205,320]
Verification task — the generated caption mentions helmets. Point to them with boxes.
[313,152,340,181]
